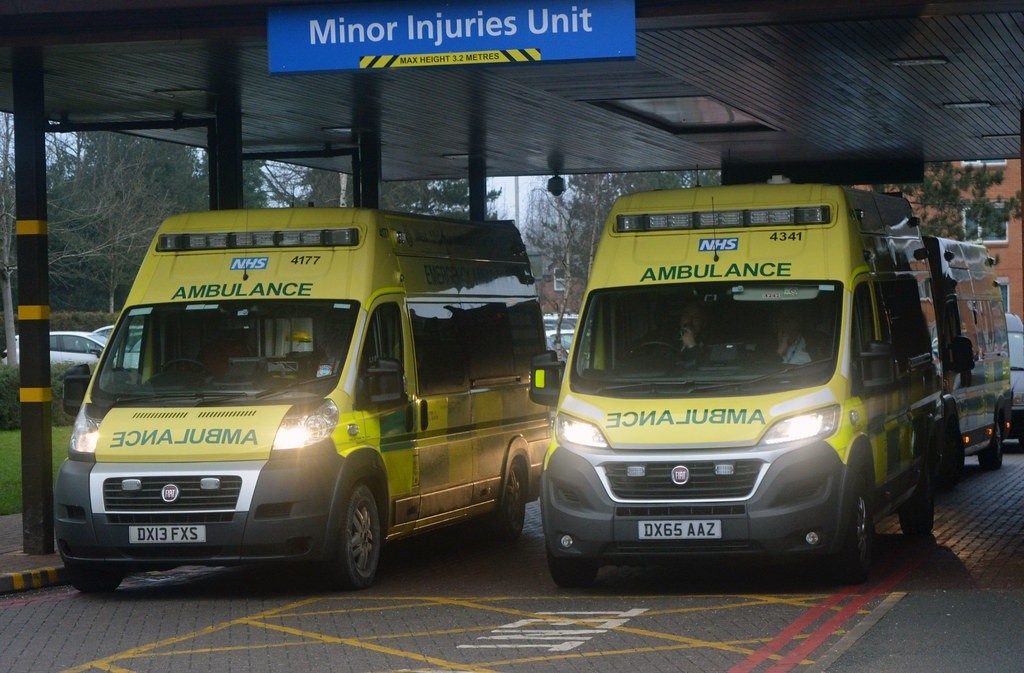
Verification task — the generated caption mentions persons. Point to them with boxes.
[626,297,711,369]
[756,299,828,368]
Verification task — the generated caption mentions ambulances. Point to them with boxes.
[528,182,950,589]
[53,205,551,595]
[920,235,1013,484]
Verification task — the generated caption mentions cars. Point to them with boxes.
[1004,313,1024,453]
[544,311,579,364]
[1,331,109,366]
[92,324,144,369]
